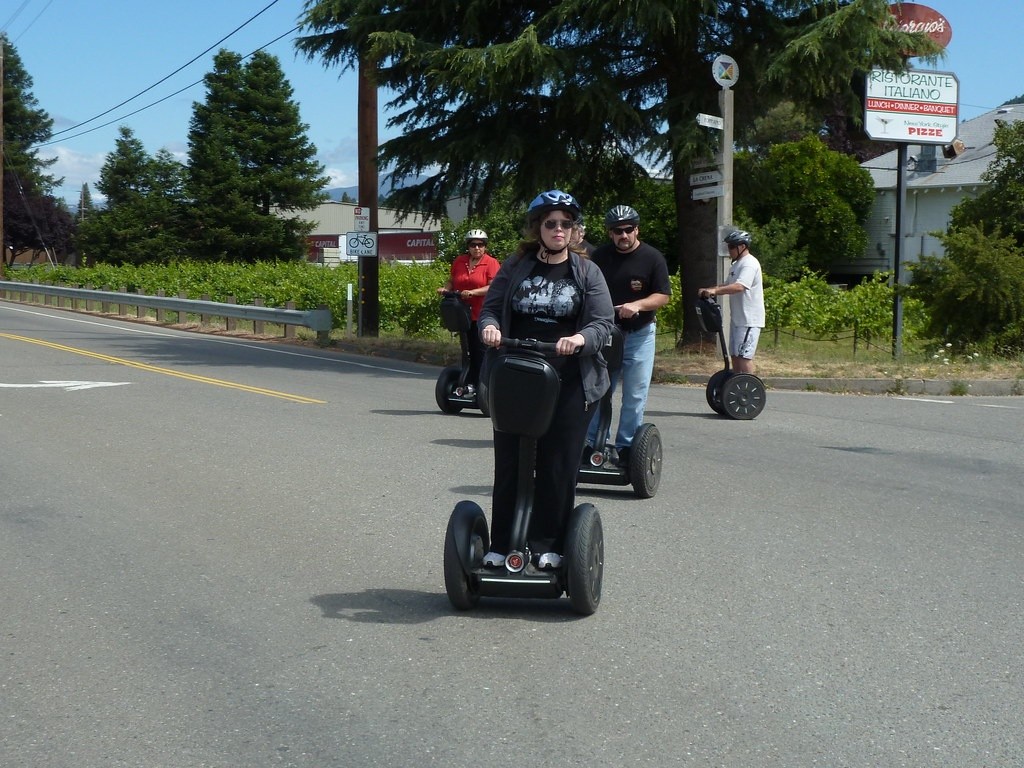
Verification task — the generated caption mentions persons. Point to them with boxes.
[580,205,672,470]
[697,230,765,377]
[436,228,501,399]
[477,190,616,573]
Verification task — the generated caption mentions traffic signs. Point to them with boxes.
[692,185,724,201]
[689,170,724,187]
[695,112,724,130]
[690,153,724,170]
[353,207,369,231]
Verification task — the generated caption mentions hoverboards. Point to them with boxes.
[444,333,604,618]
[433,289,484,414]
[575,305,663,499]
[695,291,767,420]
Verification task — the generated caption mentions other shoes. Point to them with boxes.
[581,445,594,464]
[456,384,476,399]
[615,447,631,468]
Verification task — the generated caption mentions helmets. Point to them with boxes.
[466,229,488,242]
[528,190,581,222]
[606,205,640,228]
[724,230,749,247]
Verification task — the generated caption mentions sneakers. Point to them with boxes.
[539,552,560,569]
[483,552,505,568]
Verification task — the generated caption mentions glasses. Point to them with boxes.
[468,243,485,248]
[609,226,635,235]
[728,243,744,249]
[537,219,574,229]
